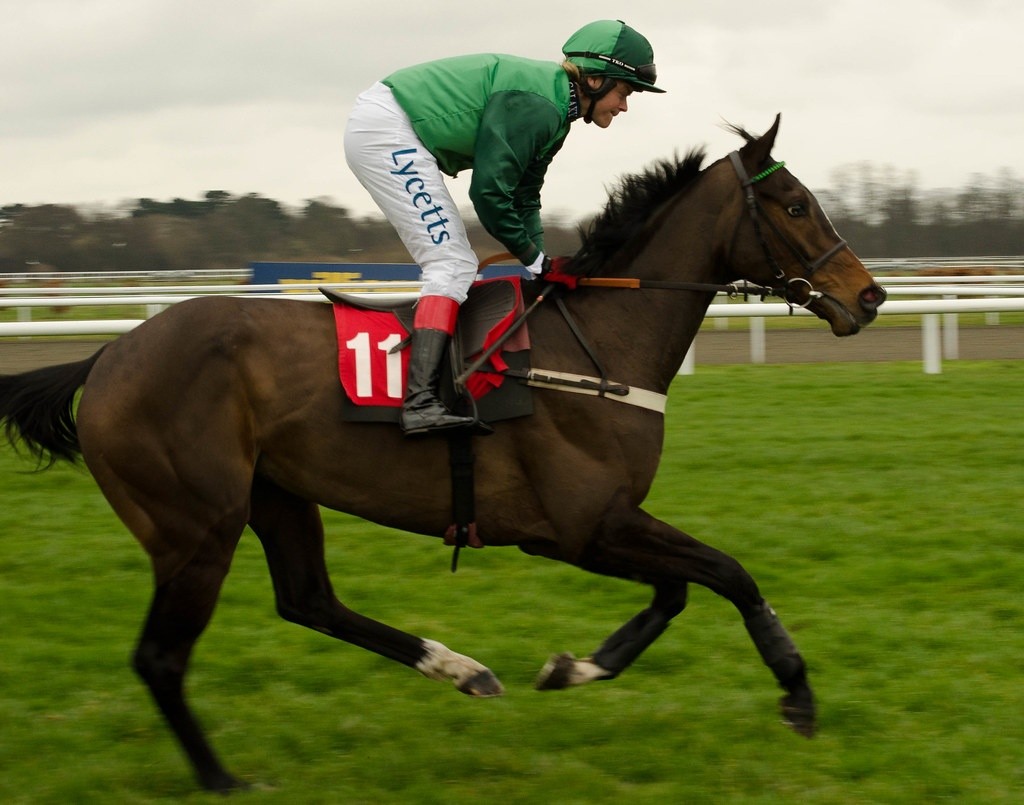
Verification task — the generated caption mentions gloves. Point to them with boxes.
[535,255,587,291]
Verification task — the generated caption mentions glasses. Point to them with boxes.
[635,63,657,85]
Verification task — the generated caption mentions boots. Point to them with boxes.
[399,328,474,440]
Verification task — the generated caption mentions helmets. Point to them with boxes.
[562,19,667,94]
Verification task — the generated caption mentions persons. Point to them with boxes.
[342,19,666,438]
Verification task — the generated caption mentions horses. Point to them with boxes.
[0,111,888,791]
